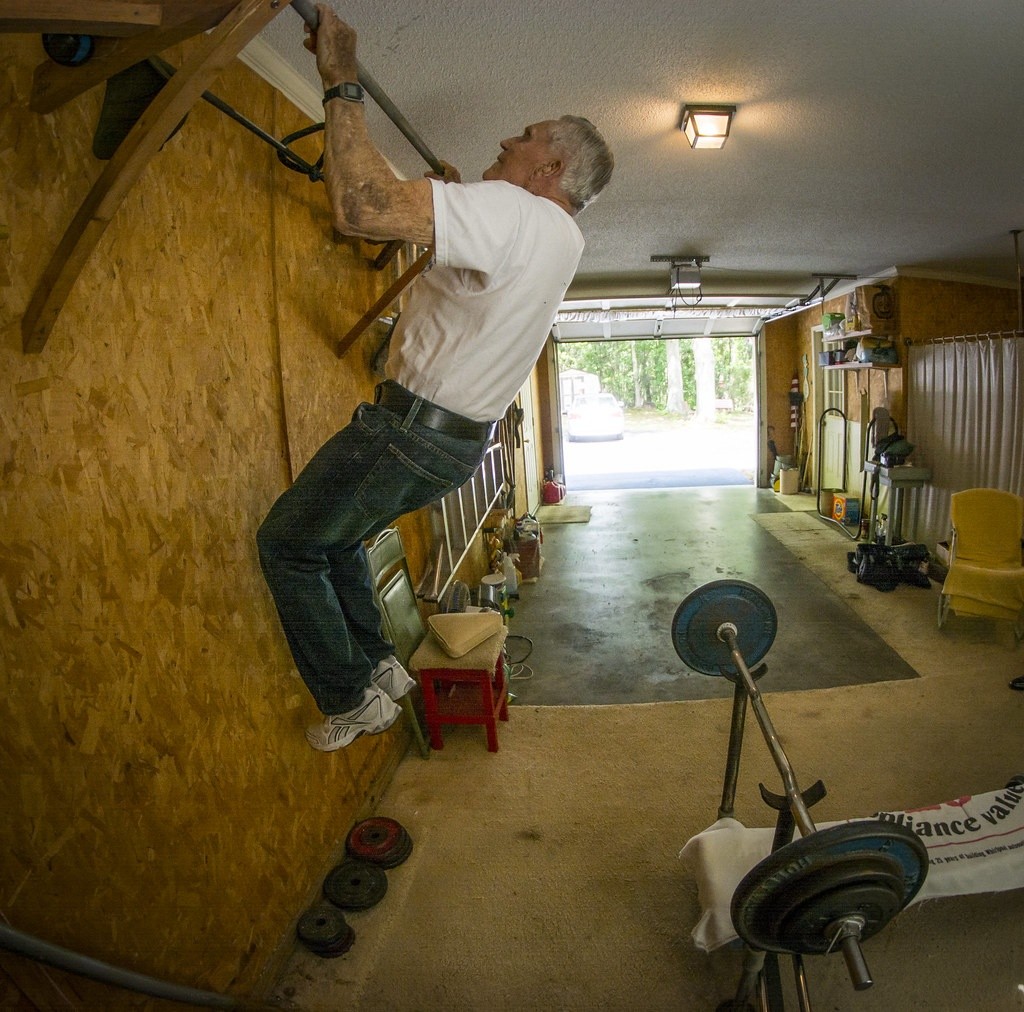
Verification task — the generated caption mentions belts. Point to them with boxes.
[371,382,496,441]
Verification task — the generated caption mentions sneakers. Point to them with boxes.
[305,681,402,752]
[369,655,417,702]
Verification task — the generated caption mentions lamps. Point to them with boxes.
[679,105,737,149]
[650,256,709,289]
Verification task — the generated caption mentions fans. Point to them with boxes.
[440,579,471,613]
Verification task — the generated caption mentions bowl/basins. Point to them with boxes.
[776,455,792,463]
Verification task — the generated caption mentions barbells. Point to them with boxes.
[670,578,930,992]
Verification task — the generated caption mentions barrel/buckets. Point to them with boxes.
[821,487,844,517]
[774,460,792,477]
[780,468,799,494]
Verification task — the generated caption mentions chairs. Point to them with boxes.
[937,487,1024,641]
[365,525,430,760]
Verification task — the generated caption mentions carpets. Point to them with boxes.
[534,505,592,523]
[768,488,820,512]
[265,658,1024,1012]
[747,512,1023,677]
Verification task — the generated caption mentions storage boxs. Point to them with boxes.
[819,352,833,366]
[508,534,540,578]
[833,493,860,525]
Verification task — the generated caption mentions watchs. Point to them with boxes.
[322,82,365,106]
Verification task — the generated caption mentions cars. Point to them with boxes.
[563,393,627,442]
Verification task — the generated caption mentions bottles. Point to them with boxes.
[888,336,897,363]
[544,480,566,503]
[886,451,894,468]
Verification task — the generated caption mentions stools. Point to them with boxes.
[408,628,509,752]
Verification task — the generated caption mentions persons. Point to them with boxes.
[255,1,615,753]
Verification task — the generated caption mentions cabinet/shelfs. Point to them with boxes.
[820,329,901,369]
